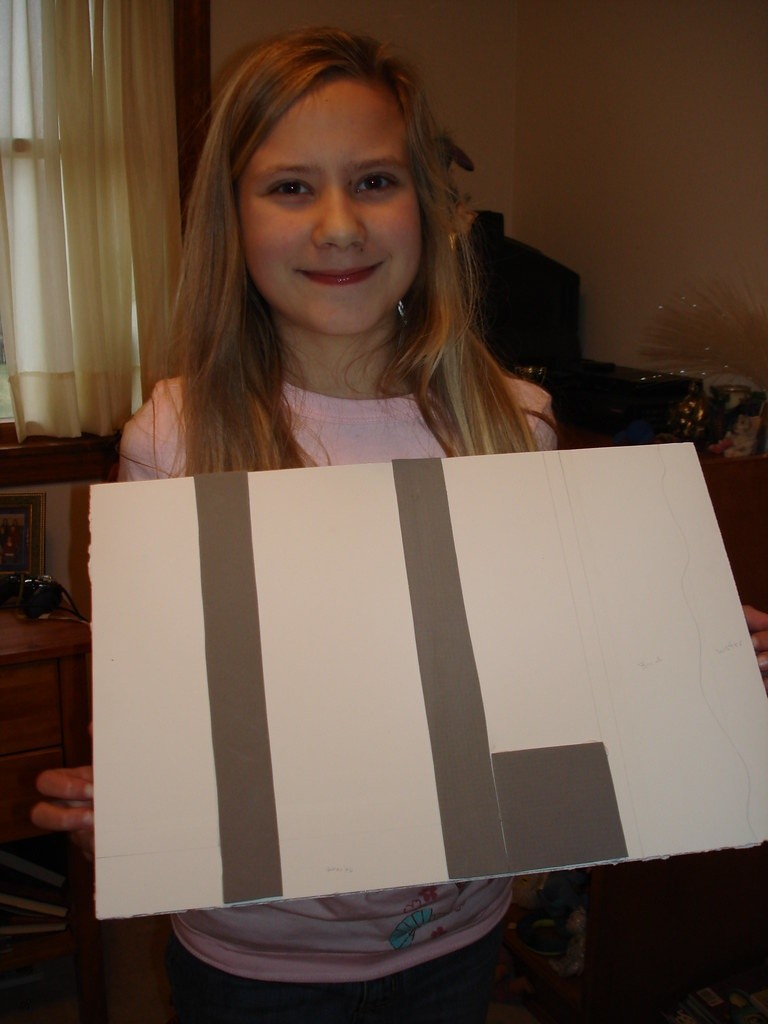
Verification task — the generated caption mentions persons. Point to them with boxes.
[393,136,584,424]
[29,32,768,1024]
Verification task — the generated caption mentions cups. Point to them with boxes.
[514,365,548,386]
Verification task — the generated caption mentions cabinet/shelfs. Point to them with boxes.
[0,603,106,1024]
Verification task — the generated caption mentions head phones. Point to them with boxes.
[2,571,63,618]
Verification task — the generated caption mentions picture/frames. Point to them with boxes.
[0,491,48,609]
